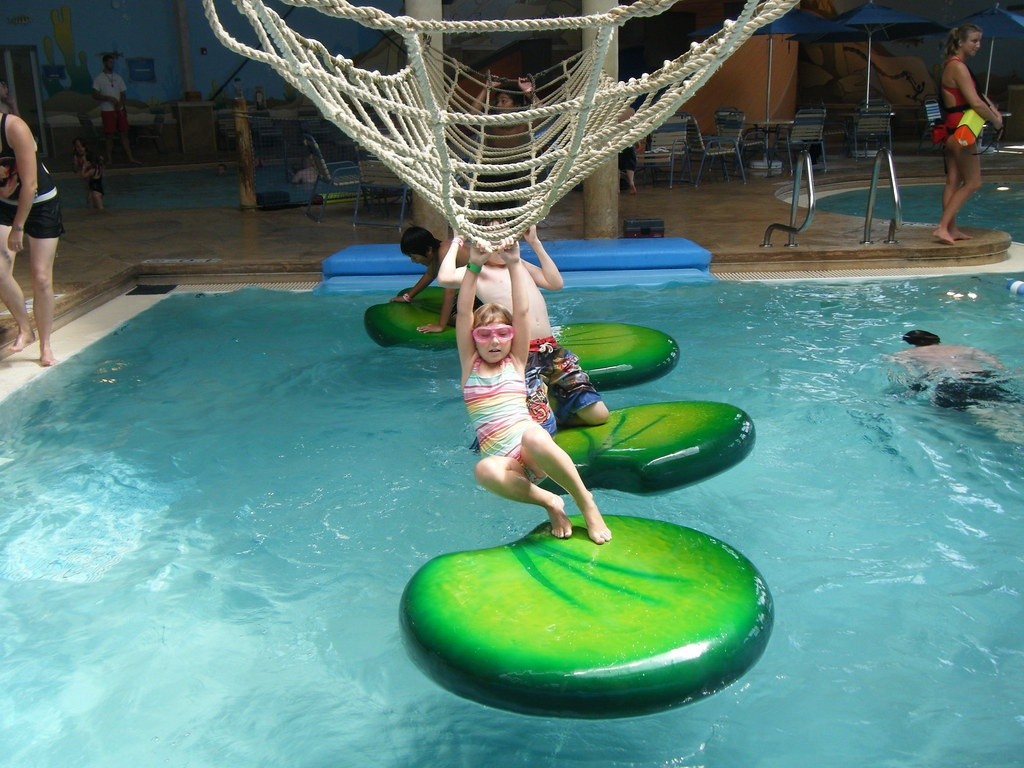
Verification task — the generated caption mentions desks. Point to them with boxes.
[835,111,896,116]
[742,119,794,125]
[999,110,1012,116]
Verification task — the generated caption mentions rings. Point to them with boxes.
[15,246,18,248]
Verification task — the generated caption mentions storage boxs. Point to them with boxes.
[622,218,665,238]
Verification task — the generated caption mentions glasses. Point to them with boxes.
[473,325,514,341]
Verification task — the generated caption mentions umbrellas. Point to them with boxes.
[688,0,1024,145]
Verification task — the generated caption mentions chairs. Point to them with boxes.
[635,94,945,188]
[75,111,170,163]
[217,105,330,152]
[301,127,480,232]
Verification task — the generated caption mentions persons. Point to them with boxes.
[289,150,320,202]
[456,236,614,546]
[438,216,610,441]
[92,54,142,166]
[933,24,1002,245]
[470,74,551,221]
[70,136,109,211]
[216,163,227,177]
[390,225,483,334]
[618,97,644,196]
[0,80,64,367]
[902,330,976,360]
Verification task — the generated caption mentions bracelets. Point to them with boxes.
[466,259,482,275]
[403,293,412,303]
[451,236,465,247]
[12,226,24,231]
[529,94,536,102]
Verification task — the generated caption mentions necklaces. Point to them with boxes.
[103,70,114,88]
